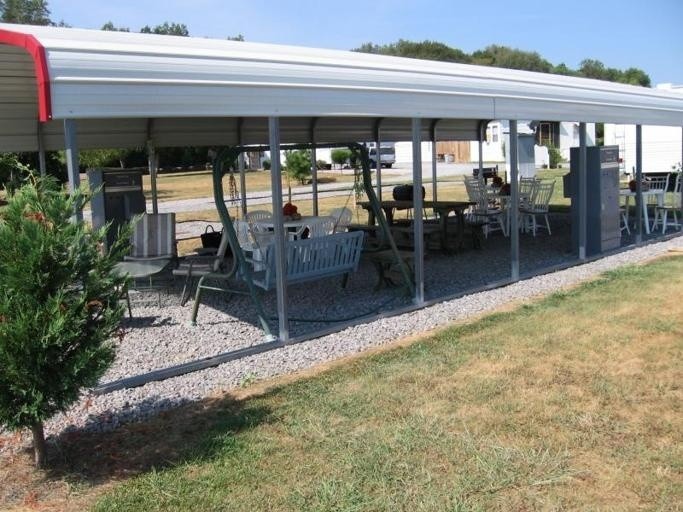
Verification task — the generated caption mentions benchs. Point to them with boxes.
[348,219,460,259]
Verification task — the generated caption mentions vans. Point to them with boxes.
[367,146,396,169]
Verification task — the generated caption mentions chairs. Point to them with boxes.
[232,206,351,272]
[462,170,556,238]
[103,212,231,308]
[634,172,682,235]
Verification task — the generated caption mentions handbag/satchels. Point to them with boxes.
[200,224,233,259]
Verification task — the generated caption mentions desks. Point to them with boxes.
[356,199,476,254]
[619,189,663,235]
